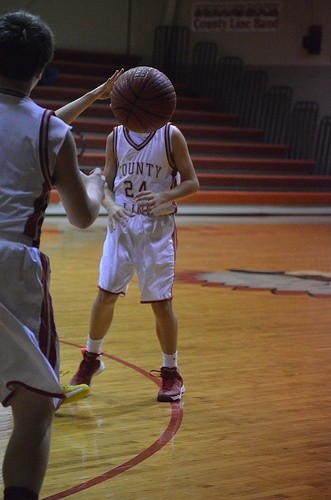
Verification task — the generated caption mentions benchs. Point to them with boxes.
[30,50,331,206]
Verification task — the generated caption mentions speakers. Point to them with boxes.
[307,26,321,54]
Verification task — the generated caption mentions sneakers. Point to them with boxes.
[156,367,185,402]
[70,349,104,391]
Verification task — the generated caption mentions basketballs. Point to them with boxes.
[109,65,177,133]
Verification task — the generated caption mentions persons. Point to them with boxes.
[69,122,200,402]
[0,10,125,500]
